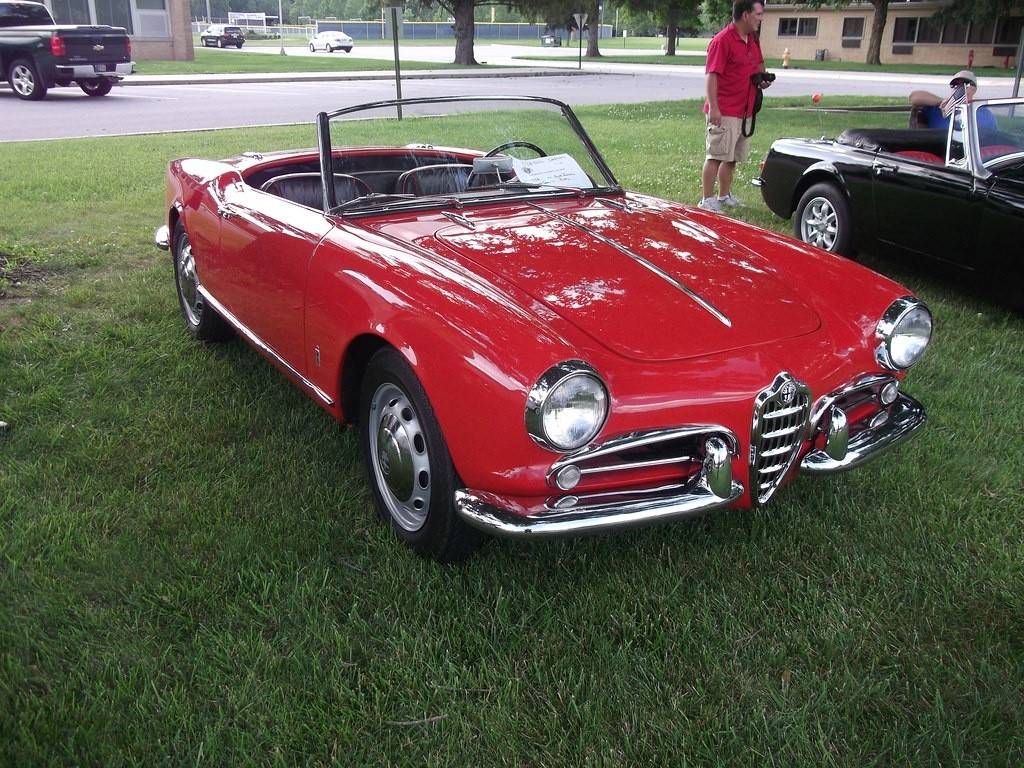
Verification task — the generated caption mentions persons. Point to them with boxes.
[908,70,998,130]
[697,0,772,215]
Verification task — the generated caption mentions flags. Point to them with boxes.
[939,83,967,118]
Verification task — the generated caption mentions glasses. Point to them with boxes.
[950,82,976,88]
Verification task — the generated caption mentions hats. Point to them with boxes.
[950,70,977,90]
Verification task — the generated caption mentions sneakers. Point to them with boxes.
[717,193,743,208]
[698,198,723,214]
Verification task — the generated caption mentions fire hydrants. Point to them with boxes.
[781,47,791,70]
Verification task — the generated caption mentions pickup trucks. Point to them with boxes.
[1,0,136,101]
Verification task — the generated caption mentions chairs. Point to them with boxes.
[980,145,1022,162]
[395,164,499,196]
[896,151,944,165]
[260,172,379,217]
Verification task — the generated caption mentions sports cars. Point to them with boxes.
[150,94,937,561]
[752,96,1024,313]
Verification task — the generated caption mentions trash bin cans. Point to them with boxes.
[815,49,824,61]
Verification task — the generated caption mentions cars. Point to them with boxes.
[306,29,353,52]
[201,23,245,49]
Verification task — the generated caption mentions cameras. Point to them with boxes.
[751,72,775,84]
